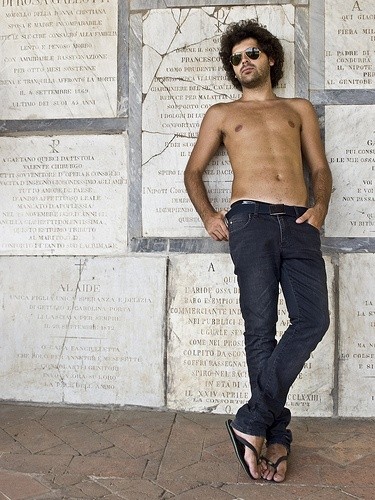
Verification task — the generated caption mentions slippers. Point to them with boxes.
[225,419,262,480]
[260,454,289,483]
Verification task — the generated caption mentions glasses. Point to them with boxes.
[229,47,261,67]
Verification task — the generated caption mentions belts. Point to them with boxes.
[225,203,309,218]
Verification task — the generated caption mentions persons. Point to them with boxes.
[185,19,330,483]
